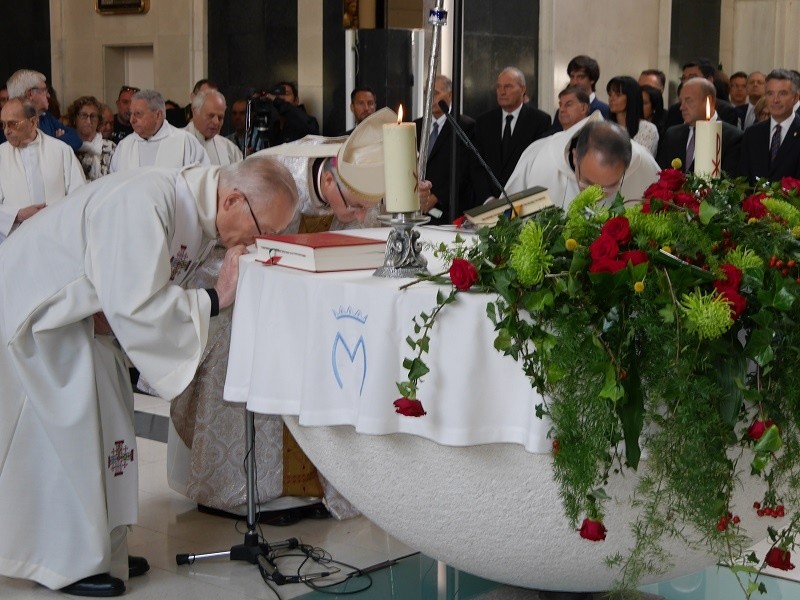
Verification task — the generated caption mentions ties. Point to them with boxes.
[503,115,513,147]
[427,122,439,159]
[771,125,782,165]
[685,128,695,170]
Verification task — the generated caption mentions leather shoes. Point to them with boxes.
[127,554,150,578]
[35,572,126,597]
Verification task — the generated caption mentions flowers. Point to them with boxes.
[385,160,800,598]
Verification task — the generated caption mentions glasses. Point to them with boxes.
[232,186,270,240]
[576,164,626,193]
[120,86,140,93]
[1,116,32,130]
[334,180,381,213]
[29,86,49,93]
[78,112,98,122]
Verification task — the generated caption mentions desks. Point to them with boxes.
[223,221,800,599]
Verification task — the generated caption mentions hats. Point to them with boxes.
[338,106,402,195]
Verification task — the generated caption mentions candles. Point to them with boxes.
[693,97,723,184]
[382,104,421,215]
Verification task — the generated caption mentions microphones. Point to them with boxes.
[438,99,503,191]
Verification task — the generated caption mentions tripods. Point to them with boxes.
[176,413,300,584]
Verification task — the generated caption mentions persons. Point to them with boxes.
[0,154,298,596]
[0,54,799,227]
[162,106,434,528]
